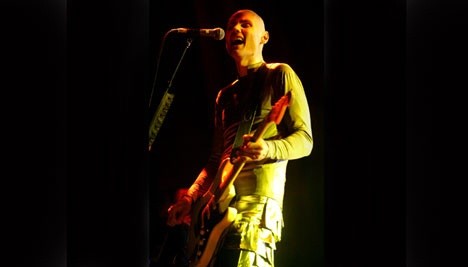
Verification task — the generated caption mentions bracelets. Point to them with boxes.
[180,195,195,208]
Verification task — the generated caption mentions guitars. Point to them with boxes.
[182,88,295,267]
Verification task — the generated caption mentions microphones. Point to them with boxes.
[178,28,225,41]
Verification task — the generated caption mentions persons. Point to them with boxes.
[167,9,314,267]
[150,183,192,267]
